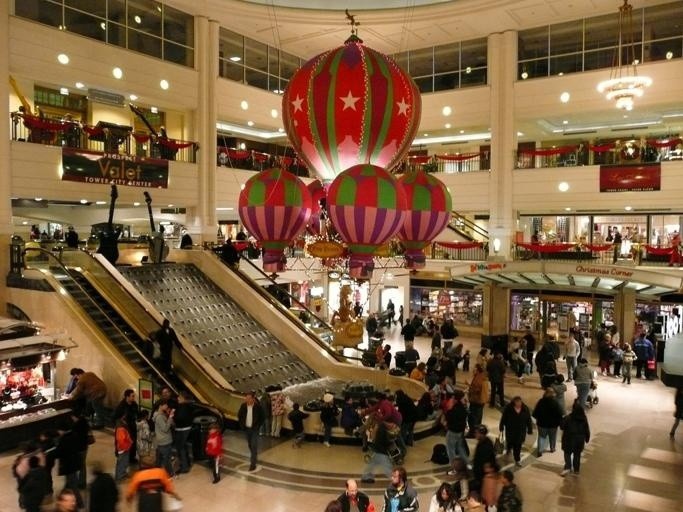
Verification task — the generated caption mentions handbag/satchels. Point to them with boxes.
[495,438,504,454]
[432,444,449,465]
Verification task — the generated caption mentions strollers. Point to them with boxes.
[584,375,594,411]
[356,414,402,468]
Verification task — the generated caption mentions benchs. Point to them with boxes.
[314,405,440,446]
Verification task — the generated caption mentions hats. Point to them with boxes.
[324,394,333,403]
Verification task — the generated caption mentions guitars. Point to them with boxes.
[129,103,178,159]
[144,191,170,262]
[9,75,58,143]
[95,185,124,262]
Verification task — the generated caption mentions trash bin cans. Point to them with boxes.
[193,416,217,460]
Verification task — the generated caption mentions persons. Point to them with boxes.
[530,230,541,260]
[668,230,683,266]
[67,225,79,249]
[31,228,60,240]
[610,226,622,264]
[212,238,238,266]
[180,227,193,249]
[669,381,683,438]
[60,112,84,147]
[236,229,245,256]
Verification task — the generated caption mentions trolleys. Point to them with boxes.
[374,310,389,330]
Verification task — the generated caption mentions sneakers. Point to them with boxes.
[516,462,522,467]
[561,469,570,477]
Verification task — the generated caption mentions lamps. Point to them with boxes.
[593,0,653,115]
